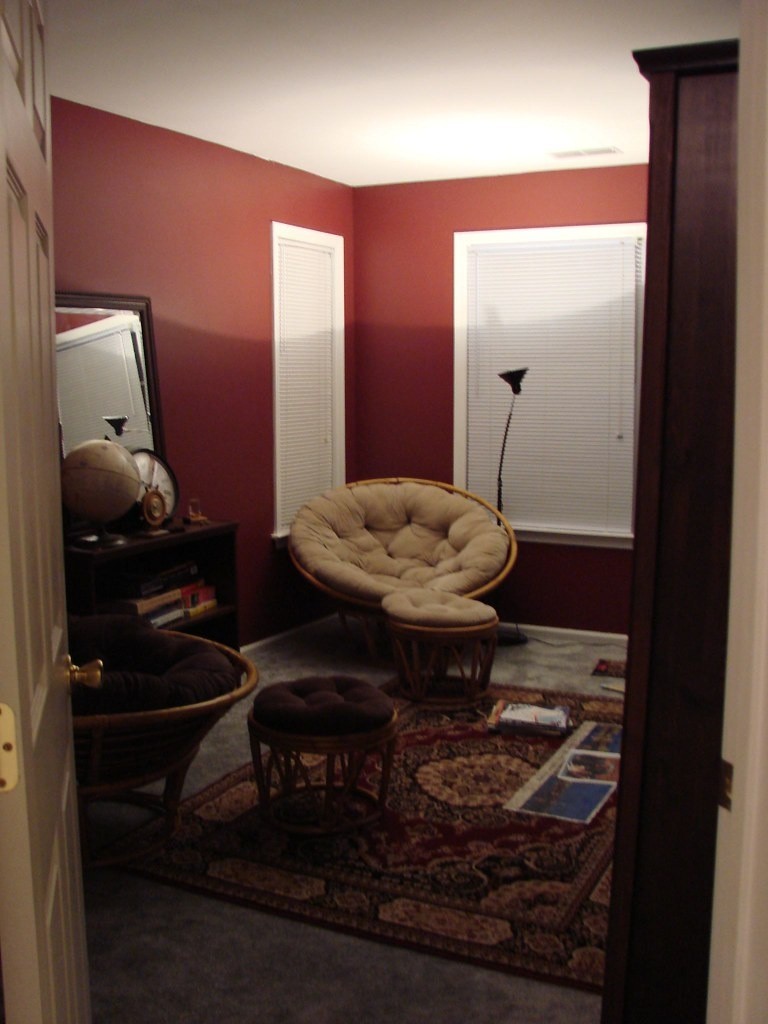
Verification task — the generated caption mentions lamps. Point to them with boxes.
[478,365,533,646]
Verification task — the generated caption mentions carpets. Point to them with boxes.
[91,647,631,992]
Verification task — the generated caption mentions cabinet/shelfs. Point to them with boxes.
[65,518,246,658]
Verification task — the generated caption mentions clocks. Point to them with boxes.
[124,447,181,527]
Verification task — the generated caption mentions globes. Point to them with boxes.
[61,439,143,548]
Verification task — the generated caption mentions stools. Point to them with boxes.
[383,586,499,708]
[67,627,259,866]
[287,473,519,656]
[247,671,402,833]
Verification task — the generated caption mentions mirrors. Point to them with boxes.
[47,290,168,472]
